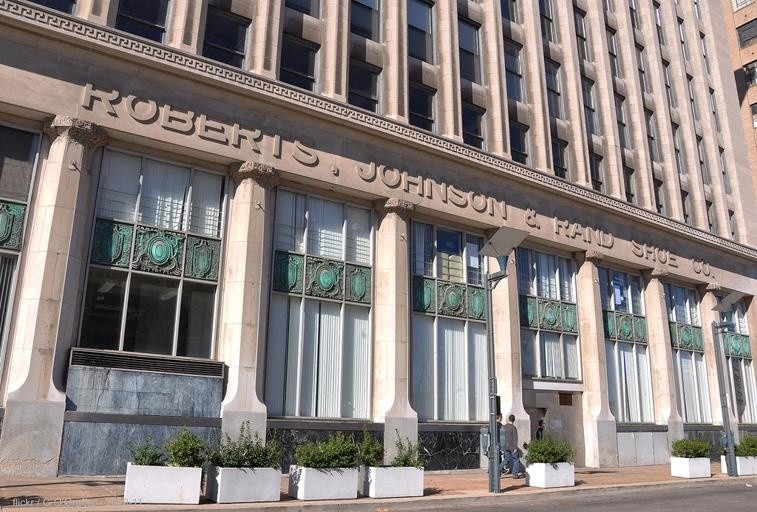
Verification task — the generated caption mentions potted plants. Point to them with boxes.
[667,431,756,479]
[524,424,576,489]
[119,423,427,505]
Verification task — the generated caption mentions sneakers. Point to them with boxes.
[513,475,519,479]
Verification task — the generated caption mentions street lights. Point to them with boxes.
[711,320,743,476]
[483,270,508,492]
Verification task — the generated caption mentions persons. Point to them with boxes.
[499,414,521,479]
[536,419,545,440]
[496,413,508,473]
